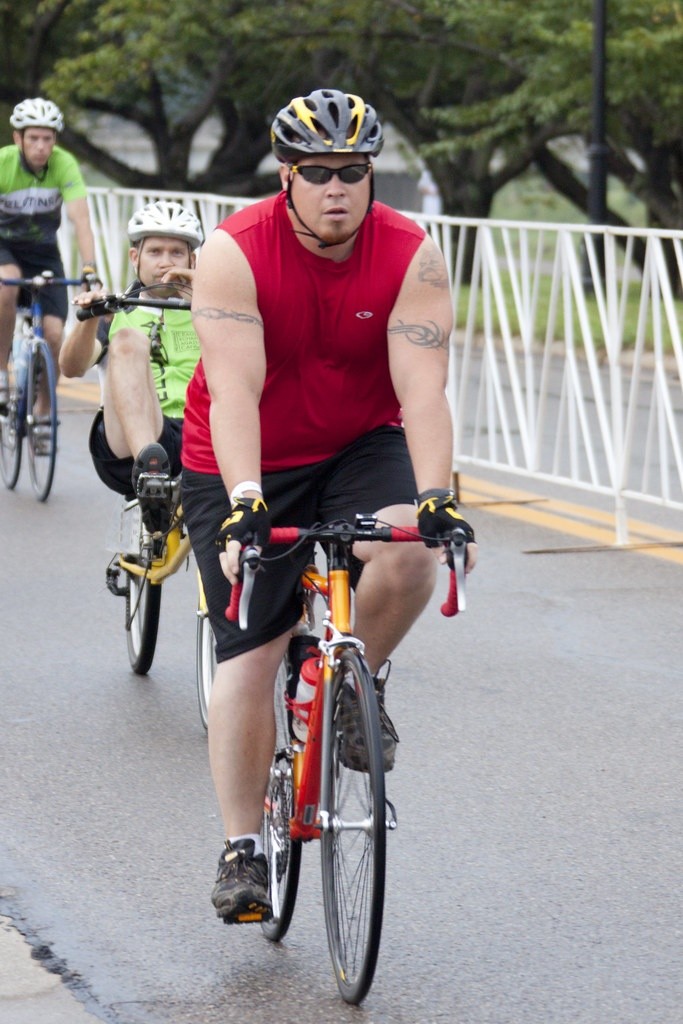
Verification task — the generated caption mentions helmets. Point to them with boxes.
[270,89,383,163]
[9,97,64,133]
[128,201,204,249]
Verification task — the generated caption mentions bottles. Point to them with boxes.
[287,635,321,736]
[292,657,319,743]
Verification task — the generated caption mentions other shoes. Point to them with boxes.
[0,375,9,404]
[32,427,52,455]
[131,443,173,531]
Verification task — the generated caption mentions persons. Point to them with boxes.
[58,201,202,534]
[0,97,103,456]
[180,89,479,925]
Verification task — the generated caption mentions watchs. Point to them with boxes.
[417,488,456,504]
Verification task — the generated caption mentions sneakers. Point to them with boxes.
[211,839,273,918]
[337,658,400,773]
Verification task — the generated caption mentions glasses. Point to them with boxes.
[290,163,372,184]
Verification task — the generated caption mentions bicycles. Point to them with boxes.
[72,281,223,733]
[0,263,96,506]
[225,515,478,1005]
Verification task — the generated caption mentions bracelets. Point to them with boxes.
[229,481,262,506]
[83,262,96,267]
